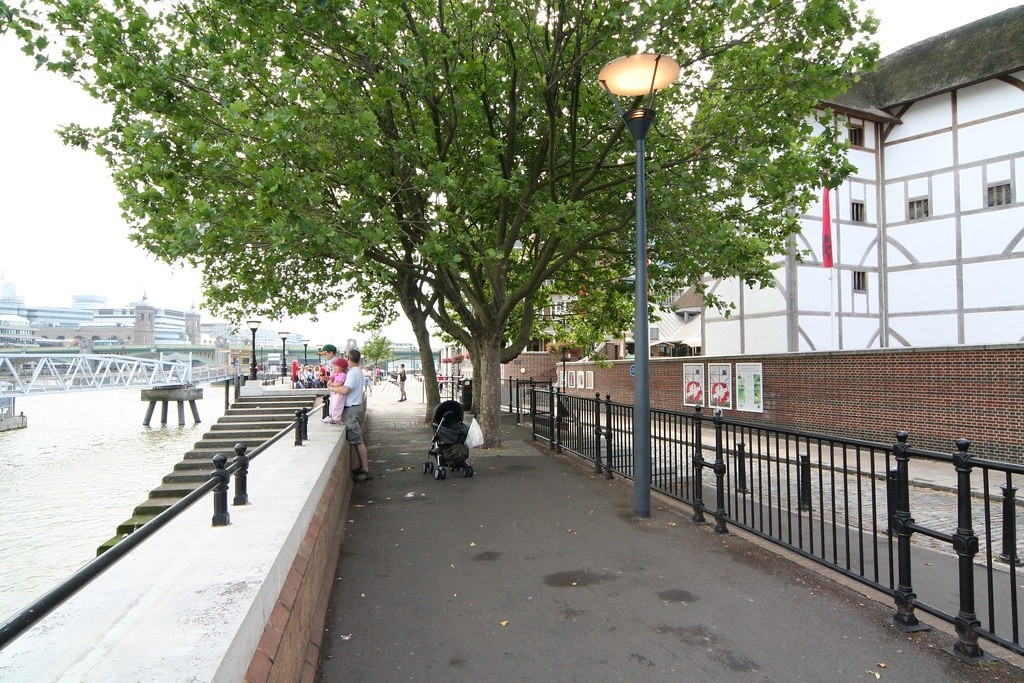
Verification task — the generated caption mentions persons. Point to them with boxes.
[363,367,372,396]
[294,363,330,388]
[373,367,381,385]
[320,359,348,423]
[396,364,407,402]
[319,344,339,382]
[328,350,371,482]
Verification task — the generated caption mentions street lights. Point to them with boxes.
[301,340,310,366]
[316,345,323,363]
[248,321,261,379]
[278,332,290,376]
[598,53,682,518]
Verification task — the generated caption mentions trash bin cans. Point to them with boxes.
[458,378,472,410]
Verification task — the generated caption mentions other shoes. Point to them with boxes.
[404,398,407,401]
[352,468,361,475]
[397,399,403,402]
[353,470,371,481]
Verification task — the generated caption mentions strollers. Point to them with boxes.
[422,399,473,479]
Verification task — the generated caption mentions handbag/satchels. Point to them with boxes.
[464,418,484,449]
[400,370,407,382]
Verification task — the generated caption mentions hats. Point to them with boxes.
[318,344,336,355]
[332,358,348,370]
